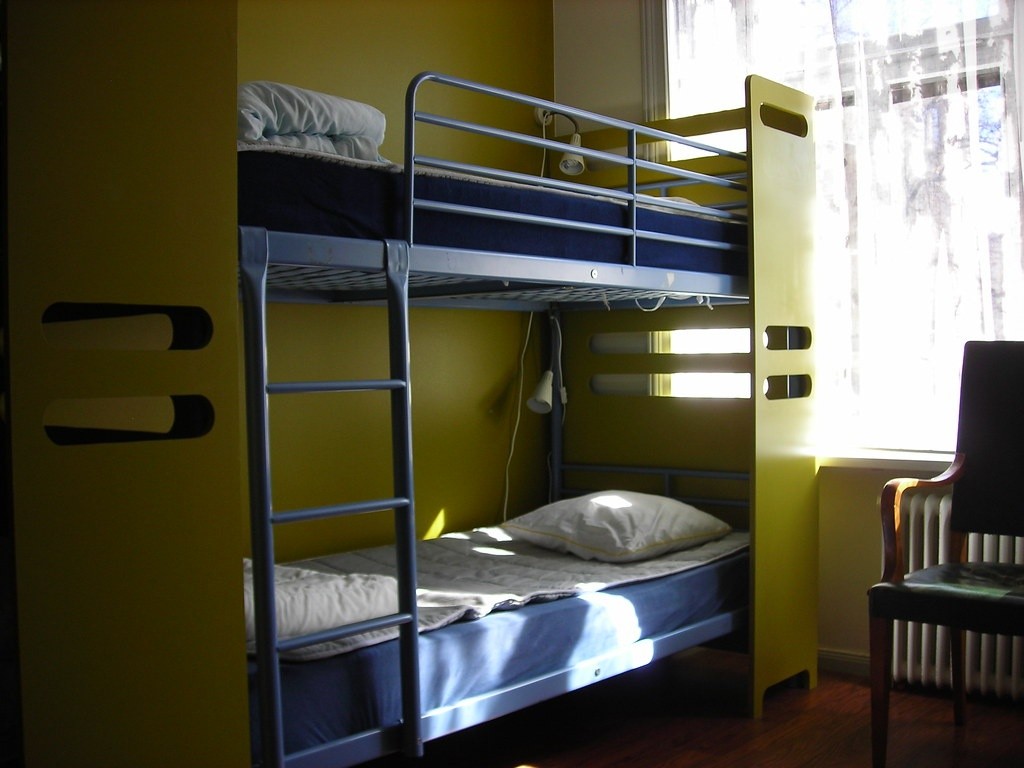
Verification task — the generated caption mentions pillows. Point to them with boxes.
[498,490,735,563]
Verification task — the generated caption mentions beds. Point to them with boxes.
[238,70,822,768]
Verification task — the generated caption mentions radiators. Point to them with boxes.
[872,487,1024,701]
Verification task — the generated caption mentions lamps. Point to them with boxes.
[525,314,561,416]
[534,105,586,177]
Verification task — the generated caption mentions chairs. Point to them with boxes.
[866,337,1024,768]
